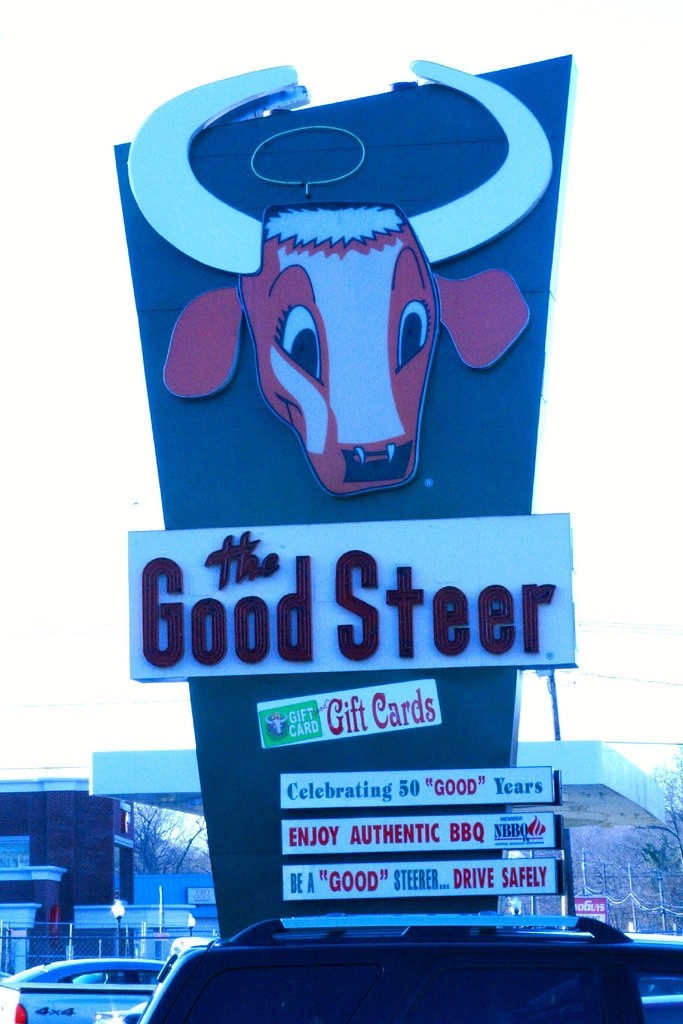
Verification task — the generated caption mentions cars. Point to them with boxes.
[1,956,167,1023]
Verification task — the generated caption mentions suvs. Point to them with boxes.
[135,914,683,1024]
